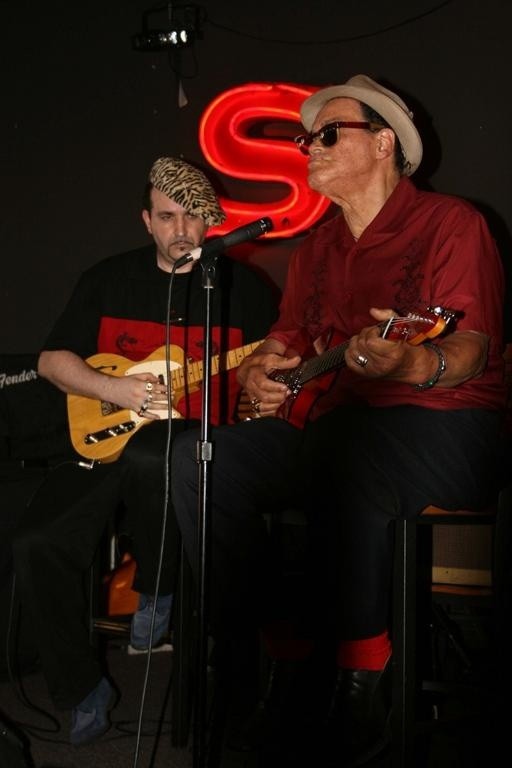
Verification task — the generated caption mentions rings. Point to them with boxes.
[141,402,148,411]
[356,354,368,366]
[250,398,261,413]
[147,389,153,399]
[145,381,152,393]
[138,411,146,417]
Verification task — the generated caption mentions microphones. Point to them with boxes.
[172,216,274,270]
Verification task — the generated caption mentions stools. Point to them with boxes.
[400,505,504,633]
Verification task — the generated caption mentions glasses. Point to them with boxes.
[295,120,384,155]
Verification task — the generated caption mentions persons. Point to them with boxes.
[32,161,283,657]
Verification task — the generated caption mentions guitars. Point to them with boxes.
[229,304,468,432]
[64,336,267,466]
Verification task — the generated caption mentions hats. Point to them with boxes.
[150,158,227,227]
[300,74,423,177]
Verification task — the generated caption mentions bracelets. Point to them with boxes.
[230,74,501,731]
[419,344,446,390]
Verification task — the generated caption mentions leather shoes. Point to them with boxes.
[337,636,393,707]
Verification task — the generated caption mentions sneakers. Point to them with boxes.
[129,587,185,650]
[69,675,120,747]
